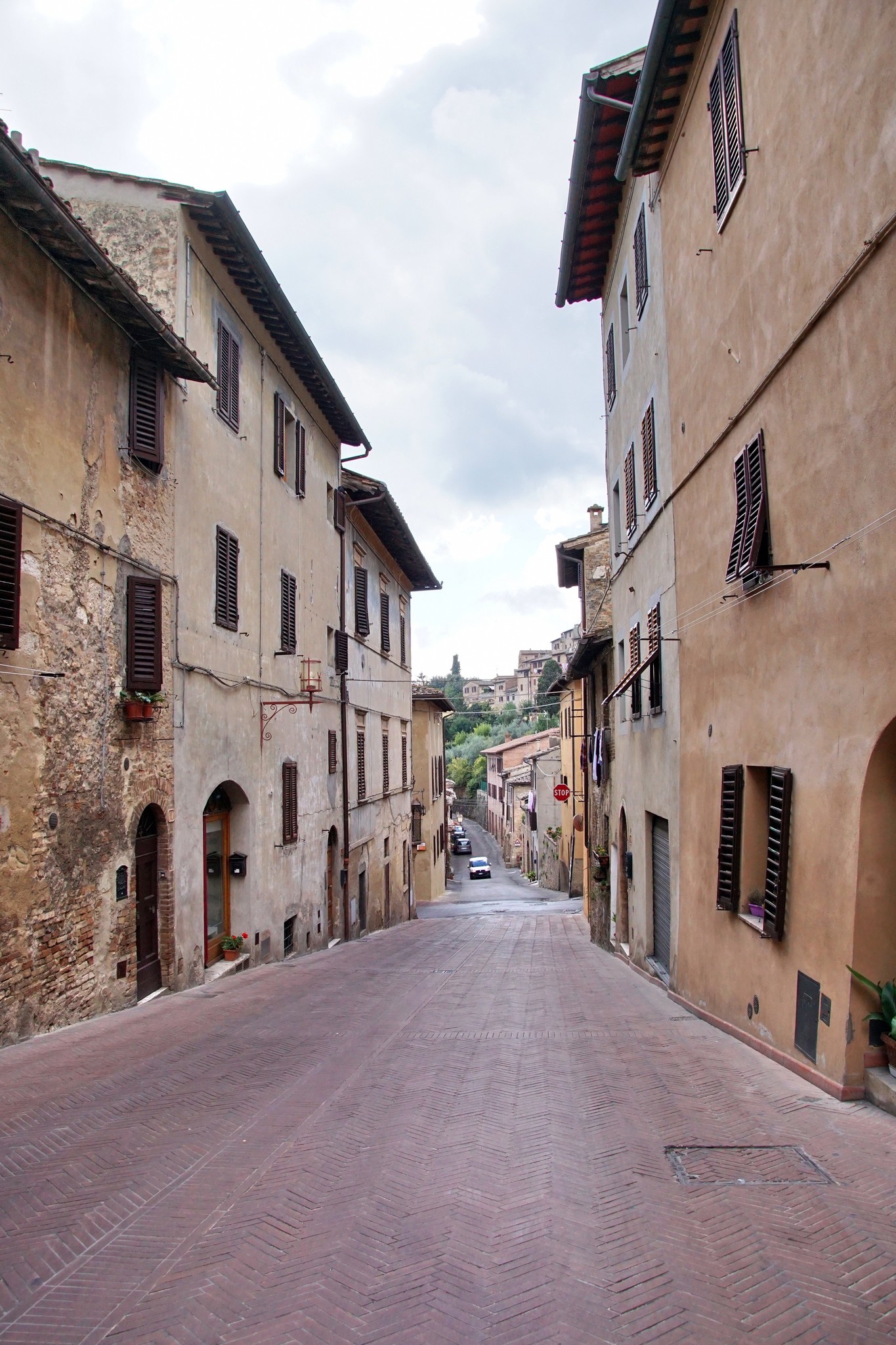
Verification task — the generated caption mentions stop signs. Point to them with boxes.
[552,784,571,801]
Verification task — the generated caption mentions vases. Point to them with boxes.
[593,850,608,865]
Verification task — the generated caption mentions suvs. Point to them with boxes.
[468,857,491,880]
[453,838,472,855]
[453,826,466,840]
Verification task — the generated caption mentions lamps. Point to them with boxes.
[412,788,426,815]
[447,814,464,825]
[260,658,323,749]
[451,812,460,819]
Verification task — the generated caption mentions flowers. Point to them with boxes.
[596,846,605,855]
[222,933,248,949]
[223,949,240,961]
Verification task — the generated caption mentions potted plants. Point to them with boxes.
[747,889,765,918]
[124,690,167,721]
[598,880,610,892]
[529,871,535,880]
[546,827,552,833]
